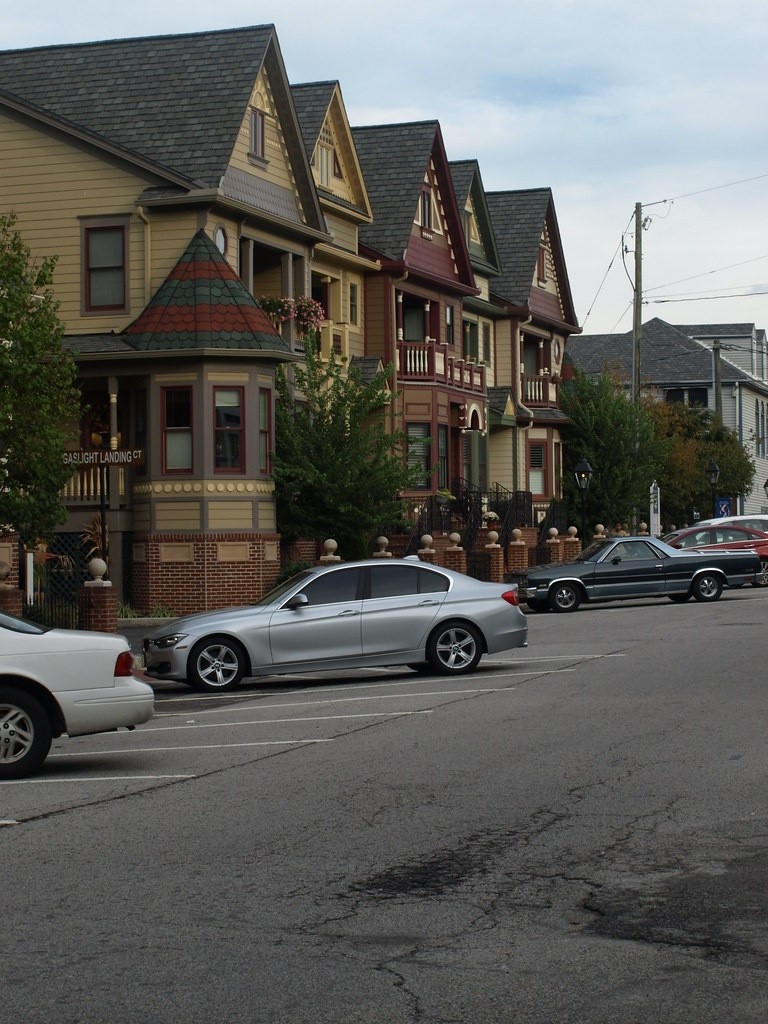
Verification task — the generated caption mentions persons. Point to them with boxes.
[611,522,627,536]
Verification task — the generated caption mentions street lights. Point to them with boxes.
[763,479,768,499]
[706,458,719,518]
[574,456,592,549]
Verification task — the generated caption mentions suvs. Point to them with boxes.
[674,514,768,547]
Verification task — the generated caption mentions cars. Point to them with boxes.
[140,559,529,693]
[504,536,764,612]
[0,610,155,779]
[623,525,768,588]
